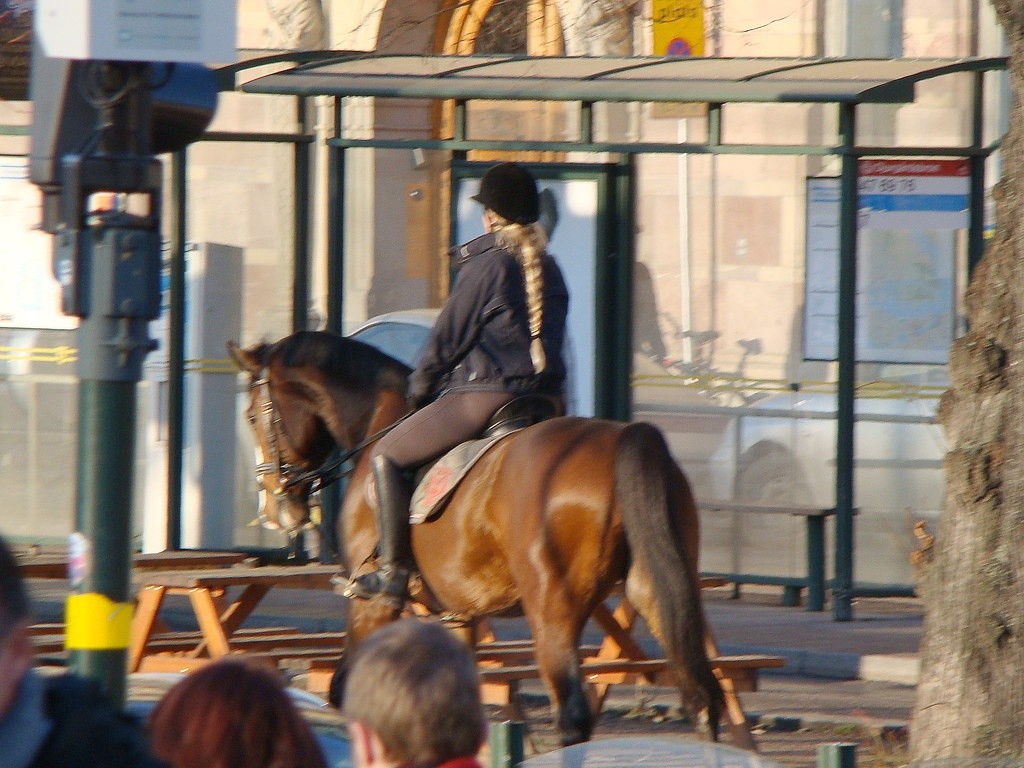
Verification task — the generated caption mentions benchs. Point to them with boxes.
[284,643,600,694]
[699,499,863,613]
[30,621,346,660]
[477,656,788,709]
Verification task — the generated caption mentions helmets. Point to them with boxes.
[471,163,540,224]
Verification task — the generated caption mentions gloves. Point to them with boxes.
[406,392,428,413]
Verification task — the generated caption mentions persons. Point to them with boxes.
[331,161,570,608]
[0,536,489,768]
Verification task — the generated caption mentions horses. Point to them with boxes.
[225,329,724,768]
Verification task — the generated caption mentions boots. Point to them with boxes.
[350,456,408,609]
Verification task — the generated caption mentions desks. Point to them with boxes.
[17,552,245,616]
[126,561,760,756]
